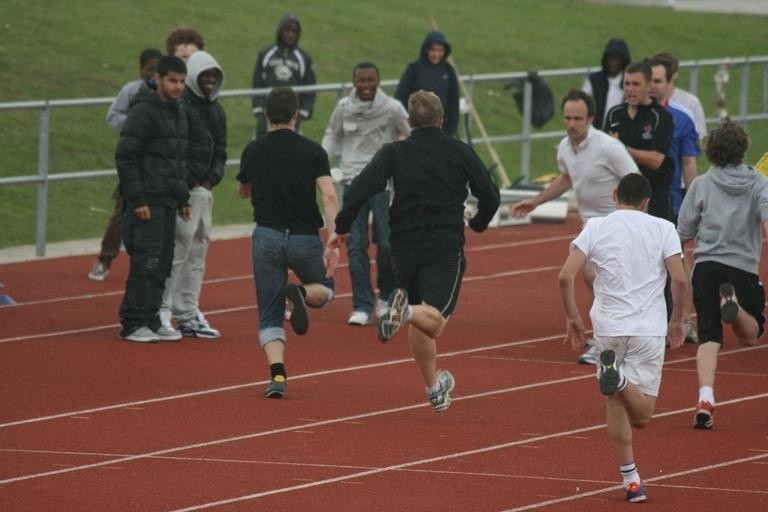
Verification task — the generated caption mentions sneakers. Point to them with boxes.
[347,310,370,327]
[684,318,698,345]
[284,283,310,337]
[153,326,183,342]
[377,288,408,345]
[719,283,739,326]
[578,344,600,365]
[599,349,620,396]
[178,319,221,339]
[693,401,714,429]
[123,327,159,343]
[89,262,110,282]
[624,478,645,503]
[426,368,455,413]
[263,380,287,399]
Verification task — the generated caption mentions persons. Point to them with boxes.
[322,63,414,328]
[160,51,228,339]
[252,11,318,137]
[118,56,189,342]
[677,117,768,428]
[165,28,206,68]
[512,90,643,297]
[582,38,630,128]
[327,90,500,412]
[664,54,707,187]
[648,60,701,189]
[398,29,457,134]
[88,49,163,281]
[560,172,687,502]
[601,62,674,220]
[237,88,336,398]
